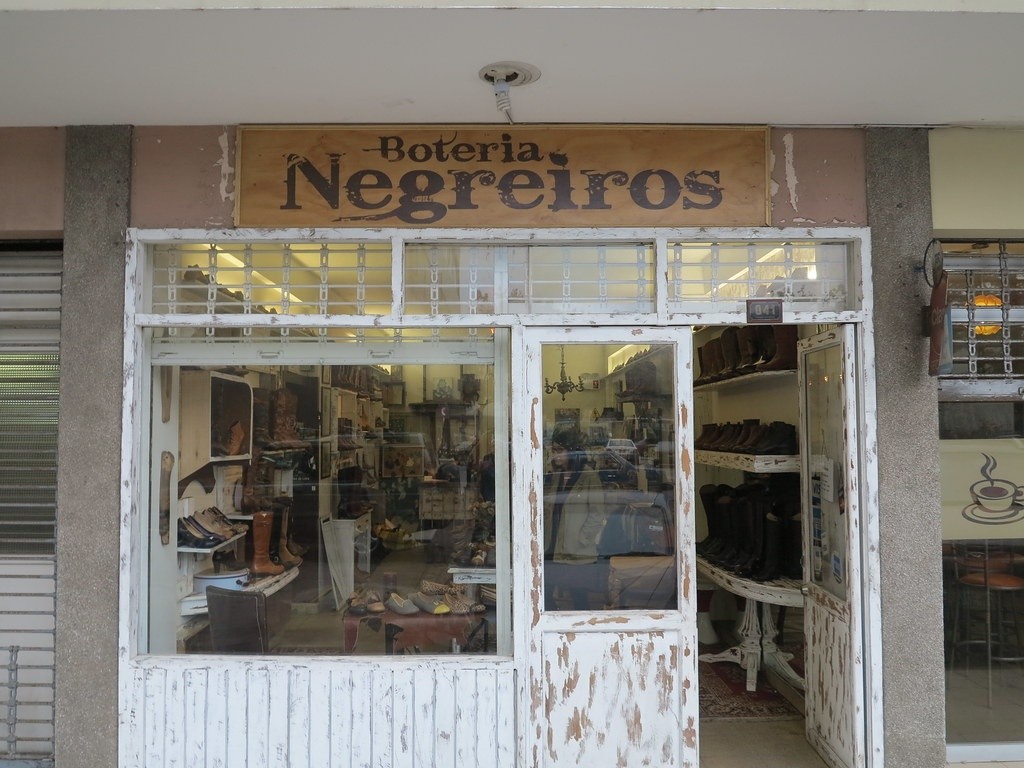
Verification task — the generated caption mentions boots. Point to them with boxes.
[338,416,364,451]
[693,326,799,388]
[331,365,384,402]
[694,483,780,582]
[253,388,312,451]
[614,361,658,397]
[248,504,304,577]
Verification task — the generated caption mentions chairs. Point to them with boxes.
[941,538,1023,709]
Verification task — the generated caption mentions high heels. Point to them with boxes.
[212,550,247,574]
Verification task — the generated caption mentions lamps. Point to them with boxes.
[544,344,585,402]
[486,68,515,112]
[961,242,1006,336]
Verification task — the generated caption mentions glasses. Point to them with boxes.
[551,447,566,454]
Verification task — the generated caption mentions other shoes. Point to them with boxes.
[365,592,385,612]
[454,590,487,612]
[695,418,797,455]
[384,593,420,614]
[346,591,367,614]
[178,506,249,548]
[421,578,467,595]
[229,420,245,455]
[338,465,374,519]
[440,593,469,614]
[408,592,450,614]
[212,424,228,455]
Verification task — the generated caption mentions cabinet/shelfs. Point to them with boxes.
[603,345,674,466]
[331,359,389,521]
[692,322,828,696]
[174,285,322,657]
